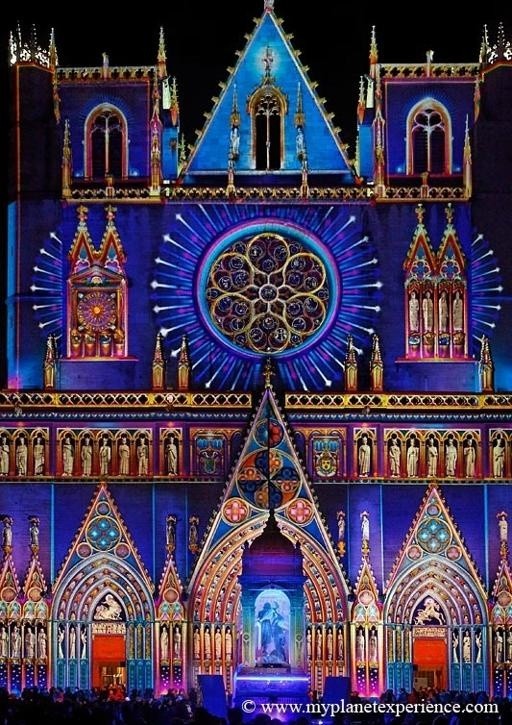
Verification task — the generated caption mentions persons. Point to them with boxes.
[54,682,512,725]
[1,120,512,666]
